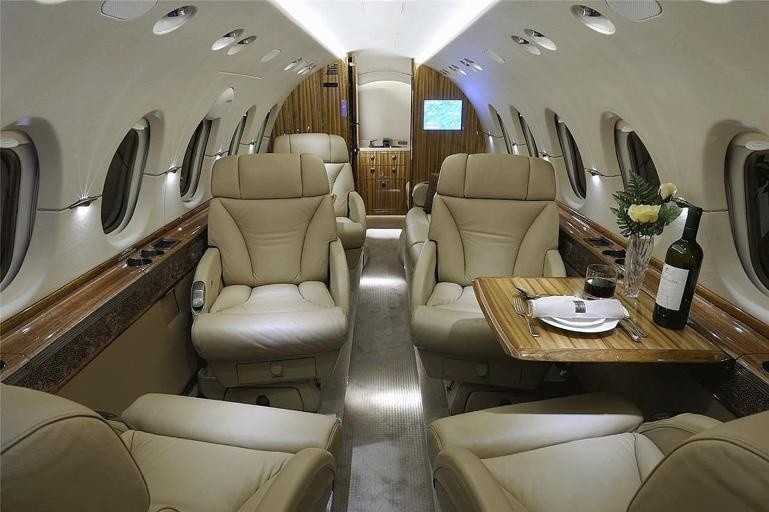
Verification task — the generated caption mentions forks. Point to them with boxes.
[511,296,539,336]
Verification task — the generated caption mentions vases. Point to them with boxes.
[612,171,684,239]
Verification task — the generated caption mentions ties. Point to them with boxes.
[423,98,462,131]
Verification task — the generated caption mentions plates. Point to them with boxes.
[357,139,410,214]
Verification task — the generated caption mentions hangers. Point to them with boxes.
[513,288,577,299]
[622,306,647,336]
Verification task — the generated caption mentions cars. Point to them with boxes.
[582,263,618,303]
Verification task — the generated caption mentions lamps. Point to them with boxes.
[274,133,366,287]
[425,388,769,512]
[1,383,345,512]
[411,154,570,413]
[400,173,439,283]
[190,153,351,413]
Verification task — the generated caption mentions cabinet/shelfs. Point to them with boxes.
[652,206,704,332]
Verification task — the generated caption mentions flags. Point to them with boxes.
[623,227,662,300]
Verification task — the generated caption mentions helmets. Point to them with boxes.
[536,295,621,335]
